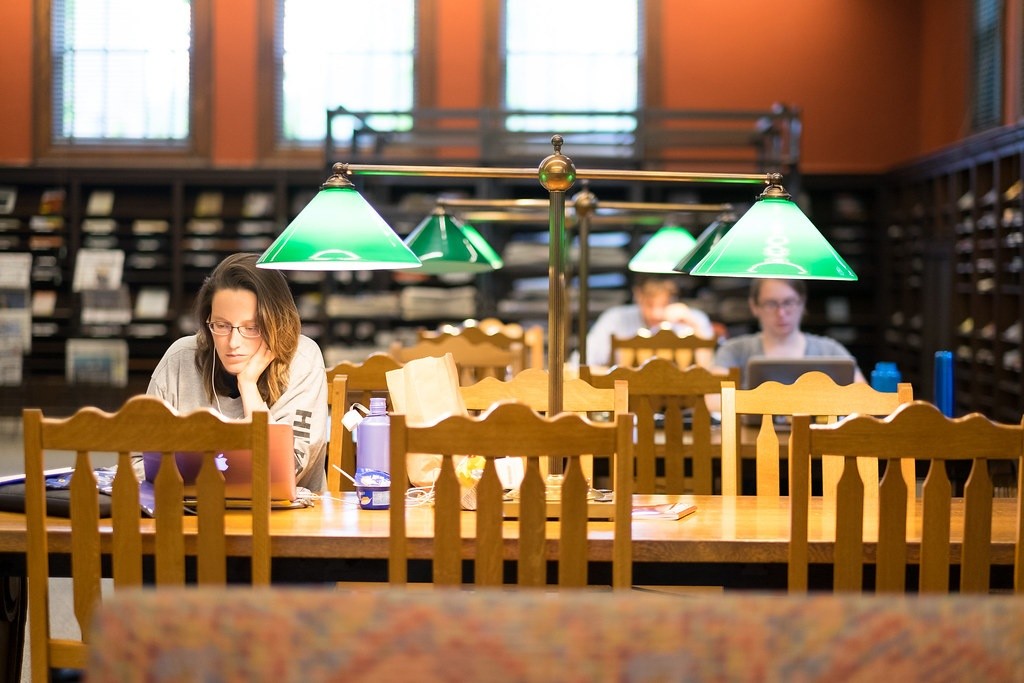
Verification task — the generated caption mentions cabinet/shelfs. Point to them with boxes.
[0,120,885,493]
[886,123,1023,481]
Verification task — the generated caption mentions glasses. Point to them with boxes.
[206,314,262,338]
[757,299,803,312]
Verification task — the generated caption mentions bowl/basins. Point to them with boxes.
[352,480,393,509]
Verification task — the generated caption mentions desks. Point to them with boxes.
[1,491,1024,599]
[627,426,812,465]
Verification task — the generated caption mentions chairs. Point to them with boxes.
[1,318,1024,595]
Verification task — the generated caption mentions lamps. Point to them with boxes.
[254,136,860,283]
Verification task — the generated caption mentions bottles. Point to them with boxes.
[871,362,899,396]
[353,398,391,472]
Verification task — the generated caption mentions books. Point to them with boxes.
[628,505,696,520]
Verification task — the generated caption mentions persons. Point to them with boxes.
[567,272,715,416]
[119,253,328,492]
[705,278,867,425]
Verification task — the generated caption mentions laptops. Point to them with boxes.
[142,422,312,509]
[737,355,856,429]
